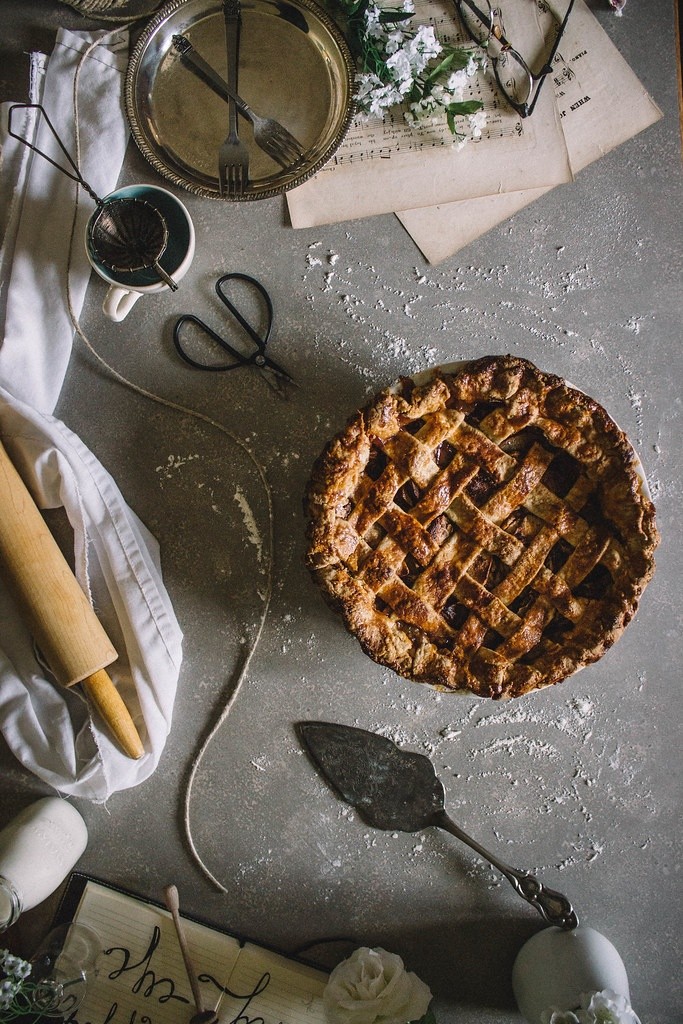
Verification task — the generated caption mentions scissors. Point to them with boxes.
[174,270,303,403]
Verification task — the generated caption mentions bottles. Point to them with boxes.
[0,796,88,935]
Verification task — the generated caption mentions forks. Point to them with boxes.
[218,0,250,198]
[171,34,306,168]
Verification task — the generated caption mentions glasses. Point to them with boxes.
[455,0,575,118]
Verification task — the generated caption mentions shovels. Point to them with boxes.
[292,719,580,934]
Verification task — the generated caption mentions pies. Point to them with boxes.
[304,353,660,701]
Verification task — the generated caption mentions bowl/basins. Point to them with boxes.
[122,0,357,202]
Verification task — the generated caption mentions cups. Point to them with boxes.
[84,183,196,322]
